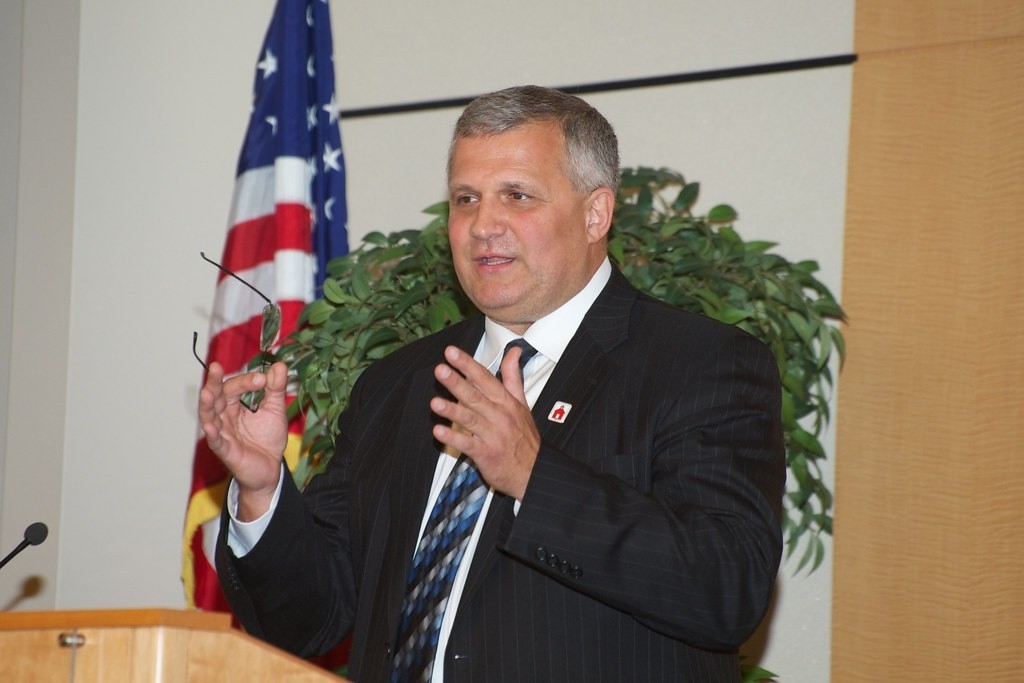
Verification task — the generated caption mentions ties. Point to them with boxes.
[389,334,537,683]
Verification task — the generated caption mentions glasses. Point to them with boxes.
[192,249,283,413]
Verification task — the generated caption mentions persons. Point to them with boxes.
[201,84,783,683]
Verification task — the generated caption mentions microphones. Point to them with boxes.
[0,522,49,570]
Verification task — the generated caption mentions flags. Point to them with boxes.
[178,1,353,616]
